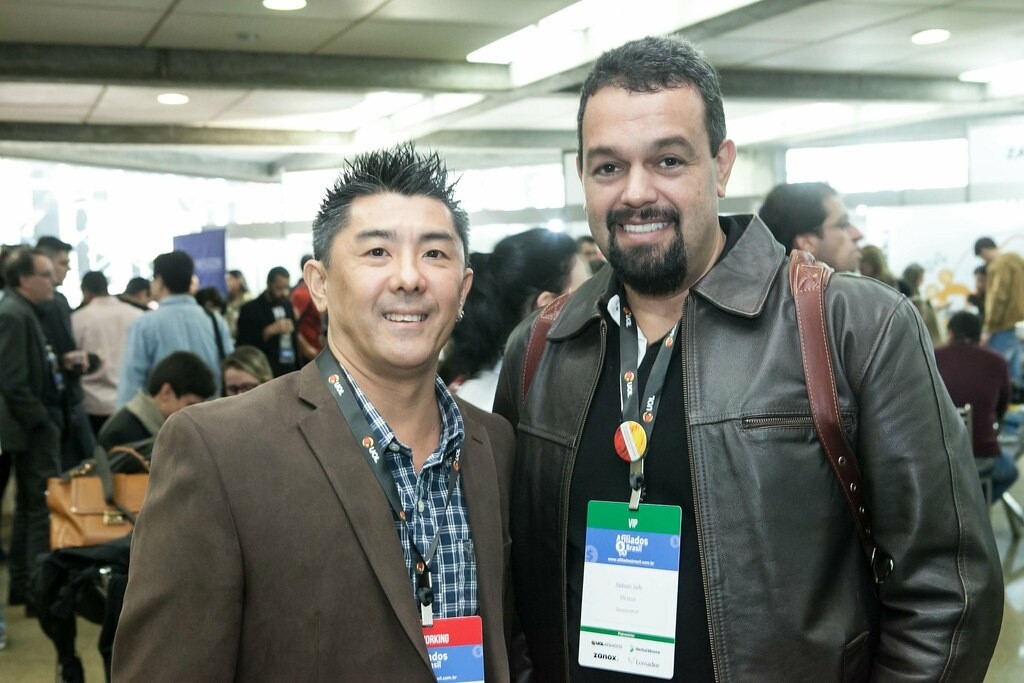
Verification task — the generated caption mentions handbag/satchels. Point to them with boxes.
[46,447,150,549]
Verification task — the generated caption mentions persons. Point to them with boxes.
[898,264,924,317]
[493,36,1004,683]
[110,143,519,683]
[965,239,1024,402]
[436,229,606,413]
[932,310,1019,506]
[859,245,898,291]
[0,235,327,683]
[758,183,863,273]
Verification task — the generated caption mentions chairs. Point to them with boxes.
[953,405,1024,538]
